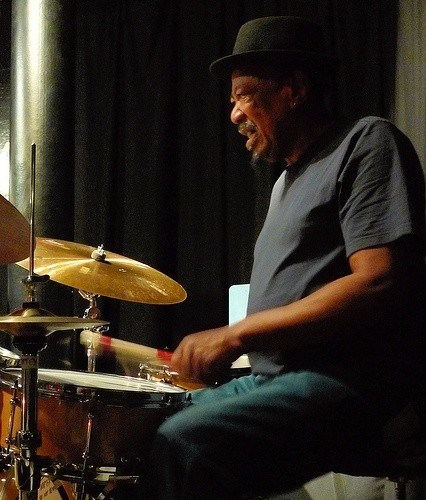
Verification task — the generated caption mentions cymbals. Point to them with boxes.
[0,309,111,337]
[16,235,186,305]
[0,193,35,265]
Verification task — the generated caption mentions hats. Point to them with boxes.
[210,16,344,79]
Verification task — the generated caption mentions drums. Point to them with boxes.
[0,367,192,485]
[0,458,86,500]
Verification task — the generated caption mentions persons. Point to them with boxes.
[118,13,426,500]
